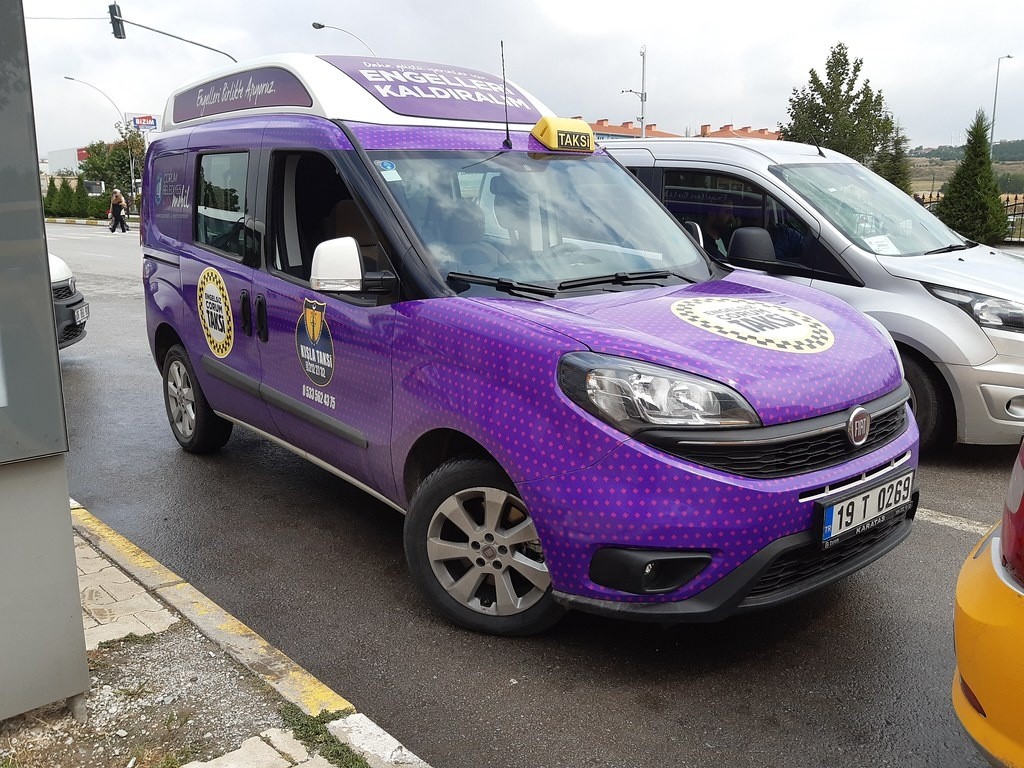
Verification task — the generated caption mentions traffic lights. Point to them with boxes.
[106,4,126,39]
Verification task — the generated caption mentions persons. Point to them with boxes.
[107,189,130,233]
[702,196,806,260]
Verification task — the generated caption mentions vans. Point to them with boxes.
[141,53,920,637]
[475,139,1024,458]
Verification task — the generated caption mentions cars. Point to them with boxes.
[926,203,1015,240]
[48,253,90,348]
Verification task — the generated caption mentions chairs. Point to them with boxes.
[433,200,508,269]
[330,195,385,299]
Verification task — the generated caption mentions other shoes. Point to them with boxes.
[109,226,114,233]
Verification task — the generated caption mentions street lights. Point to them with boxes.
[619,45,646,139]
[64,76,136,211]
[989,55,1014,160]
[313,22,377,56]
